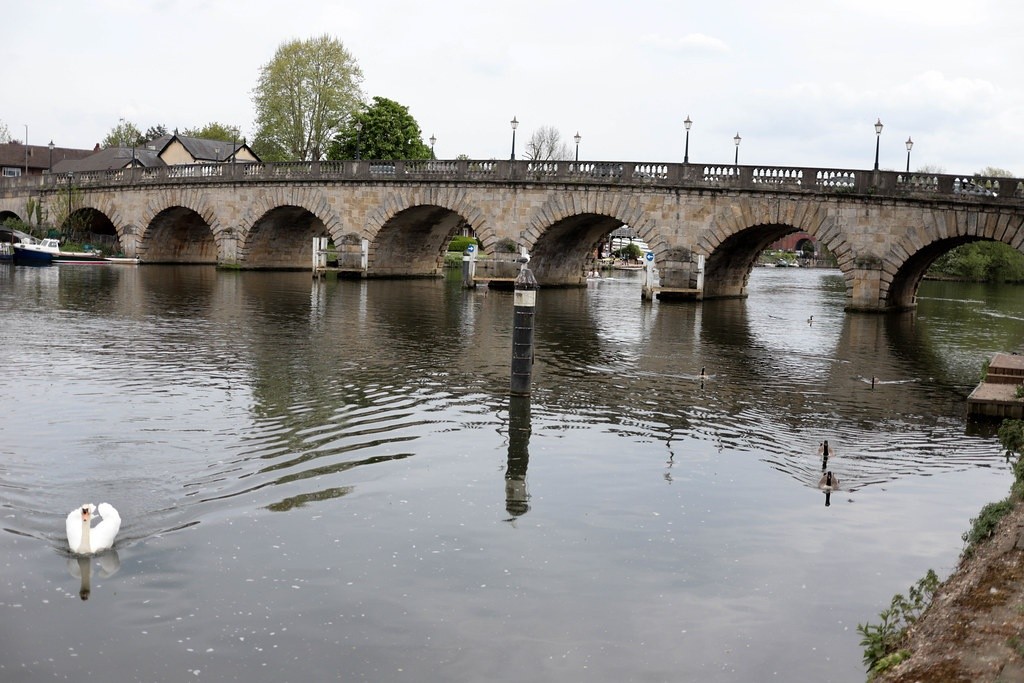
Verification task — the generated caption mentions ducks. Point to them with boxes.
[807,315,814,321]
[817,471,840,490]
[869,375,879,384]
[819,440,833,456]
[699,366,706,375]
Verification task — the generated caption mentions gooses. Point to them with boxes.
[67,547,122,601]
[65,502,122,556]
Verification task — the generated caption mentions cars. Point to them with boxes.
[933,178,998,199]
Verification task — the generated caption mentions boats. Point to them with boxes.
[12,237,65,263]
[763,256,811,268]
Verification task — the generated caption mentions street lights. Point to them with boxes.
[510,115,520,159]
[428,133,437,163]
[904,136,914,181]
[734,132,742,175]
[873,118,884,171]
[47,140,55,173]
[682,114,694,163]
[231,127,240,164]
[573,131,582,173]
[130,134,138,167]
